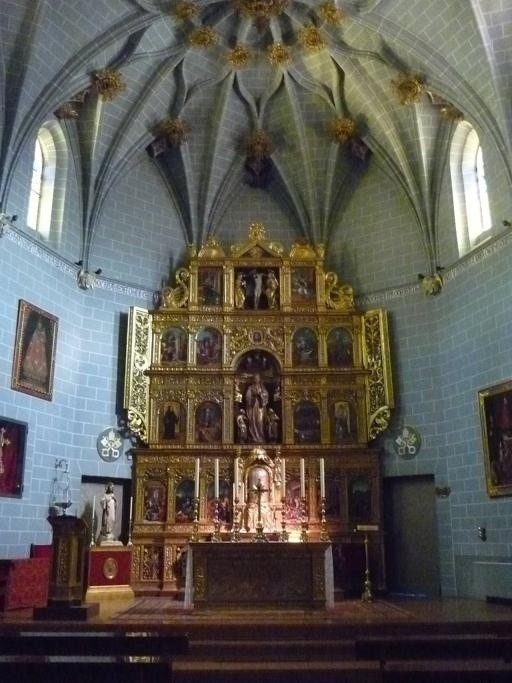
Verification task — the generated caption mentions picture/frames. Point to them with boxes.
[0,416,29,498]
[479,379,512,497]
[10,299,59,401]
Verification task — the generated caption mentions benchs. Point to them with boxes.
[354,638,512,681]
[1,636,188,683]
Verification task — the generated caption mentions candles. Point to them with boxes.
[281,459,286,498]
[300,458,306,498]
[91,495,96,520]
[214,457,219,498]
[129,496,133,524]
[233,457,238,497]
[194,458,200,498]
[320,458,326,498]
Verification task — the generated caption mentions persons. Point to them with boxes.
[235,407,252,443]
[265,408,280,444]
[243,371,270,444]
[100,482,118,537]
[162,405,181,437]
[252,268,264,310]
[234,270,247,308]
[265,268,279,309]
